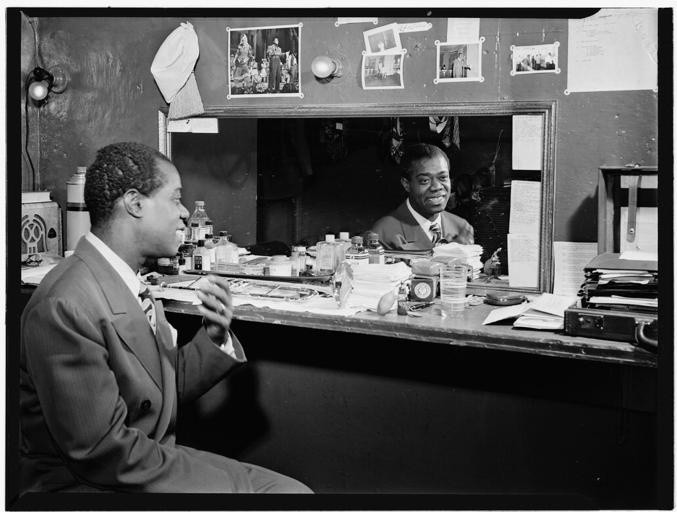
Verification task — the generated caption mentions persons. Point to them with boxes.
[232,32,255,93]
[518,50,556,73]
[266,34,291,93]
[379,63,387,80]
[377,38,385,52]
[18,141,318,495]
[451,47,466,77]
[355,141,475,268]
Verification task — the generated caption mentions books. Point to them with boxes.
[480,292,581,331]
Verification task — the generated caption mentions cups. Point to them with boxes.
[439,265,468,317]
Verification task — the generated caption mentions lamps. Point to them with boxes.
[28,64,69,100]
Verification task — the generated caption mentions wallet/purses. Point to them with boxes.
[483,293,526,305]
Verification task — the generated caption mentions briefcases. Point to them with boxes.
[564,166,658,350]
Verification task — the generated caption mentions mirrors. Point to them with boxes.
[158,101,557,294]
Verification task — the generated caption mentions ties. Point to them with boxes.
[429,222,440,244]
[139,281,157,335]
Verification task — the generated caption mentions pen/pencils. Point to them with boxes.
[410,302,434,311]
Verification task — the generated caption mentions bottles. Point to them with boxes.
[335,231,352,263]
[171,201,242,265]
[344,235,368,265]
[157,238,212,276]
[64,164,95,253]
[366,231,385,265]
[315,234,344,277]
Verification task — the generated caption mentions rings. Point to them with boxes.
[217,302,225,314]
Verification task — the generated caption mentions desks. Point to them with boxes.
[16,242,659,371]
